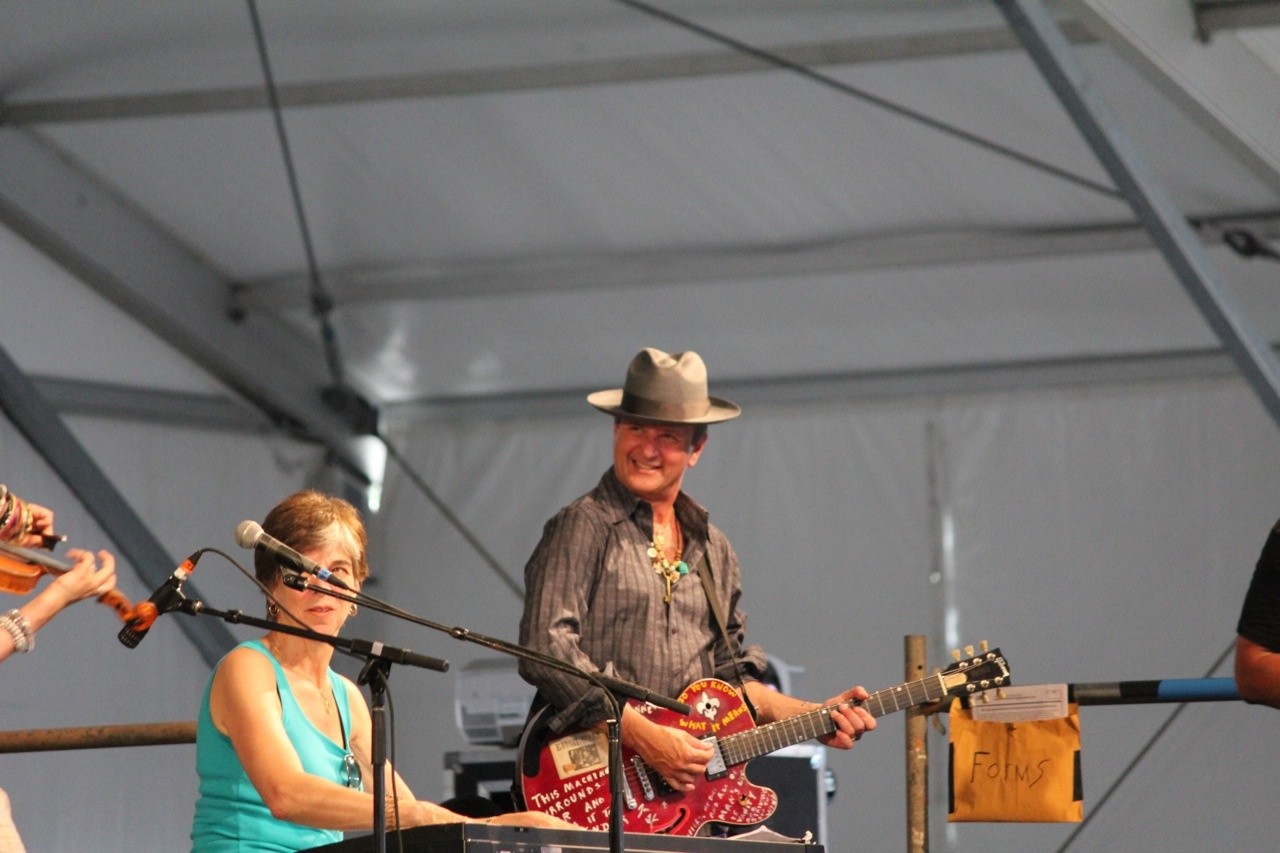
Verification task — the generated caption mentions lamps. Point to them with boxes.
[300,383,390,514]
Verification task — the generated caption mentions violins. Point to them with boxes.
[0,541,157,632]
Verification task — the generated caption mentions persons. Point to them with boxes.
[0,500,117,664]
[517,347,877,793]
[189,491,579,853]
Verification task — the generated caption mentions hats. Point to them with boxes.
[587,344,744,424]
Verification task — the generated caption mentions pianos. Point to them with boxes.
[295,822,825,853]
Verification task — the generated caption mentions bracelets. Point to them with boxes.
[0,607,35,654]
[0,484,33,547]
[385,793,396,833]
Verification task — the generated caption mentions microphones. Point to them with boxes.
[118,549,202,649]
[234,520,349,590]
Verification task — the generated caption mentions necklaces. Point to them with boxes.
[649,519,681,604]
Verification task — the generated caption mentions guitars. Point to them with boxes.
[519,638,1013,835]
